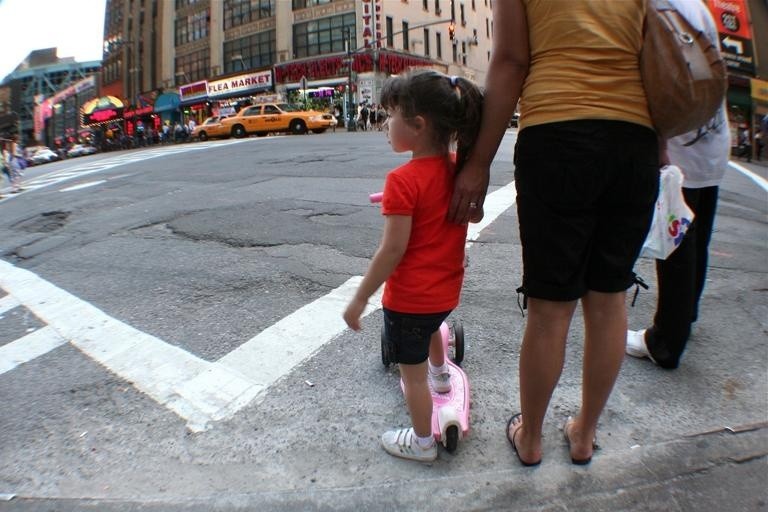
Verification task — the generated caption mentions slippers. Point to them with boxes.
[563,416,596,465]
[506,413,543,466]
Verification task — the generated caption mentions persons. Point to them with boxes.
[341,70,484,464]
[301,101,387,132]
[735,127,753,163]
[445,3,675,468]
[623,0,733,377]
[104,119,196,149]
[2,143,14,185]
[0,151,8,199]
[10,134,26,194]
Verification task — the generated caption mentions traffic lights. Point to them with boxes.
[449,26,455,40]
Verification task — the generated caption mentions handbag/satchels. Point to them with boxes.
[640,0,727,143]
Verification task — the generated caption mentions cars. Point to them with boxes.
[221,102,337,137]
[32,149,58,161]
[192,113,237,141]
[67,144,96,157]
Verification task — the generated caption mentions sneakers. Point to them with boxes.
[427,360,452,394]
[625,329,660,365]
[382,427,438,462]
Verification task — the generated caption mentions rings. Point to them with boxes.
[469,202,478,209]
